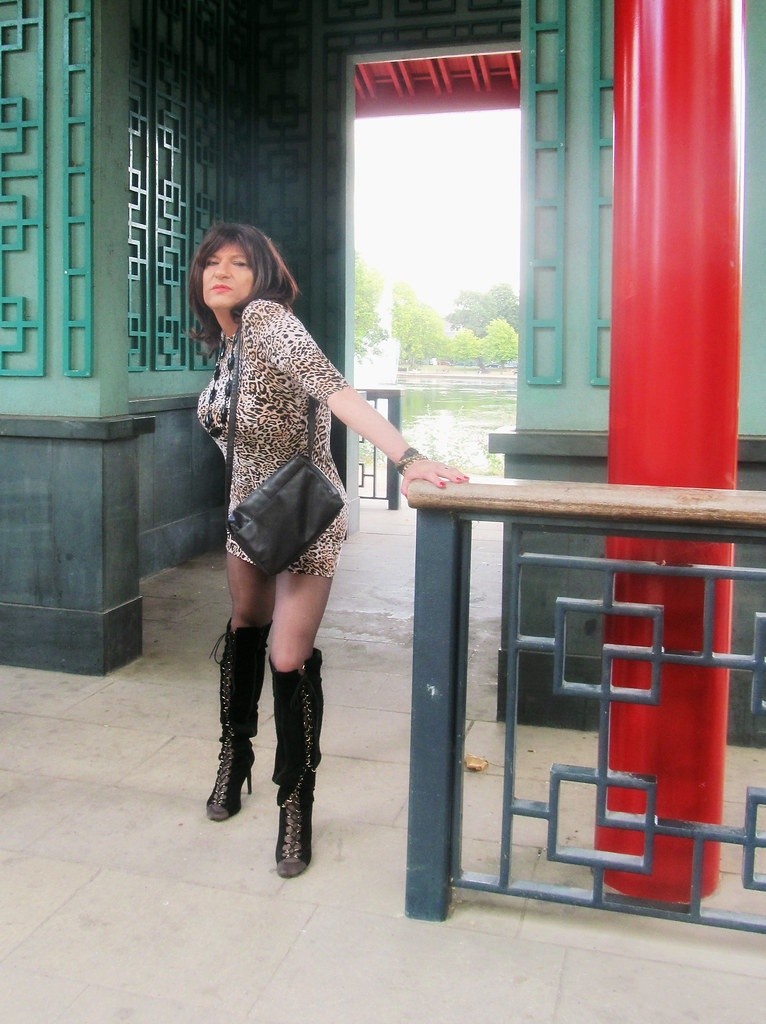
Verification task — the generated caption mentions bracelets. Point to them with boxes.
[395,448,428,477]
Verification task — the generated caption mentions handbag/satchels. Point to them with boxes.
[228,454,345,578]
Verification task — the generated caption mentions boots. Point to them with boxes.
[269,648,324,878]
[206,617,272,822]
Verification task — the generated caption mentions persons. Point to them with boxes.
[189,223,470,879]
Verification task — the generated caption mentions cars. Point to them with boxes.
[484,363,503,368]
[438,360,453,366]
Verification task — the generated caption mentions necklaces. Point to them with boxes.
[205,328,239,437]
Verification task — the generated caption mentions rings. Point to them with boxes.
[445,467,448,470]
